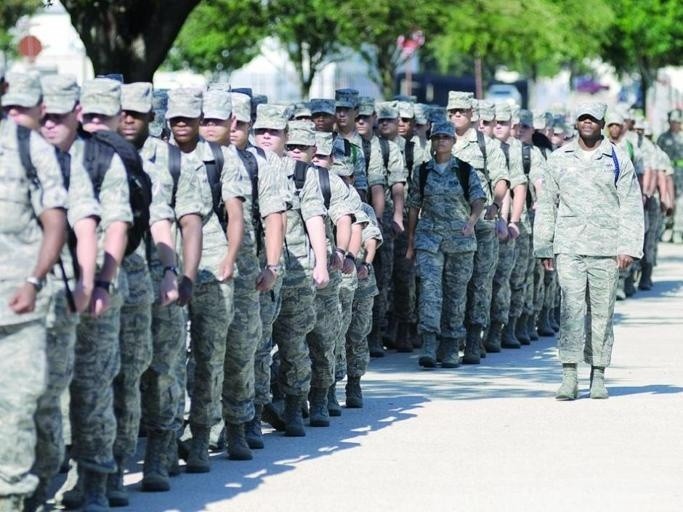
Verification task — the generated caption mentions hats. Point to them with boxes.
[429,121,455,137]
[203,91,232,120]
[252,104,287,130]
[293,88,446,124]
[232,92,251,123]
[447,91,653,138]
[41,75,80,115]
[164,87,202,119]
[316,131,333,155]
[285,120,316,146]
[0,70,42,107]
[82,77,121,116]
[121,82,153,114]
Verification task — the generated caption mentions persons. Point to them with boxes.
[533,102,645,400]
[383,89,581,367]
[3,72,245,512]
[1,52,67,512]
[208,88,408,458]
[603,108,683,301]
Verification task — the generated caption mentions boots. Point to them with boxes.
[310,376,363,427]
[262,393,308,436]
[590,366,608,398]
[615,263,652,300]
[555,363,577,399]
[539,307,560,336]
[418,324,486,367]
[486,314,539,353]
[186,424,210,472]
[63,452,129,512]
[141,430,179,491]
[225,403,264,460]
[368,322,422,357]
